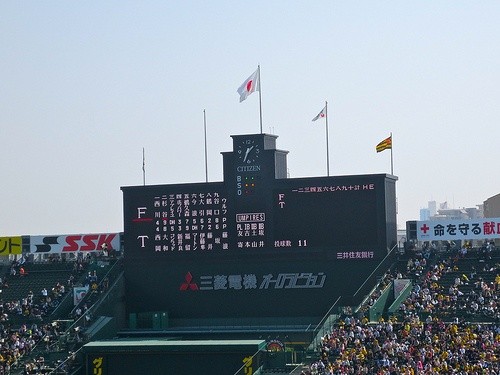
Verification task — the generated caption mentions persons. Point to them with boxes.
[0,242,122,375]
[298,237,500,375]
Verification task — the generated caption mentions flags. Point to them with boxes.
[237,67,260,103]
[311,105,327,121]
[376,136,392,153]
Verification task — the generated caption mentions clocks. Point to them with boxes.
[237,139,260,164]
